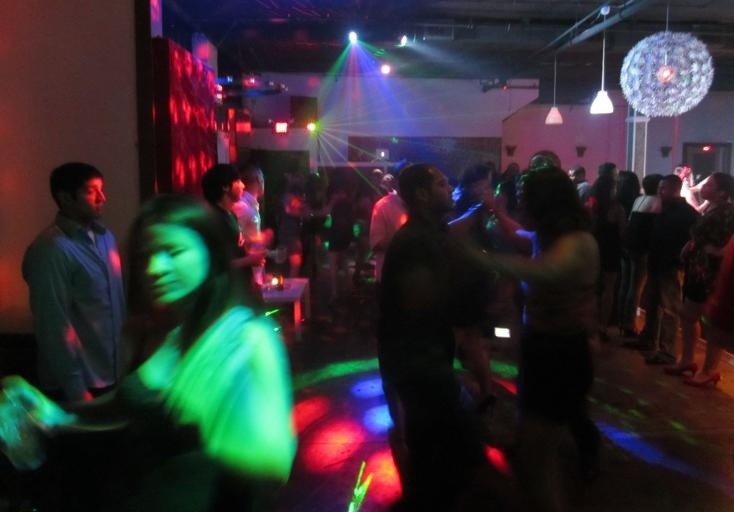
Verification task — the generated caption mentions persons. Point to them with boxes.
[1,150,733,510]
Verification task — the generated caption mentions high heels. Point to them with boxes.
[616,320,640,337]
[664,362,699,376]
[684,371,721,388]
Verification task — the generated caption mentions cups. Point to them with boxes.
[272,272,286,290]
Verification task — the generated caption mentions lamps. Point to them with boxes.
[618,0,716,118]
[589,7,614,115]
[544,54,564,125]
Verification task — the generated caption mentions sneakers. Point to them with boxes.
[645,350,676,365]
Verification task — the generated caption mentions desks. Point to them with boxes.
[262,274,310,329]
